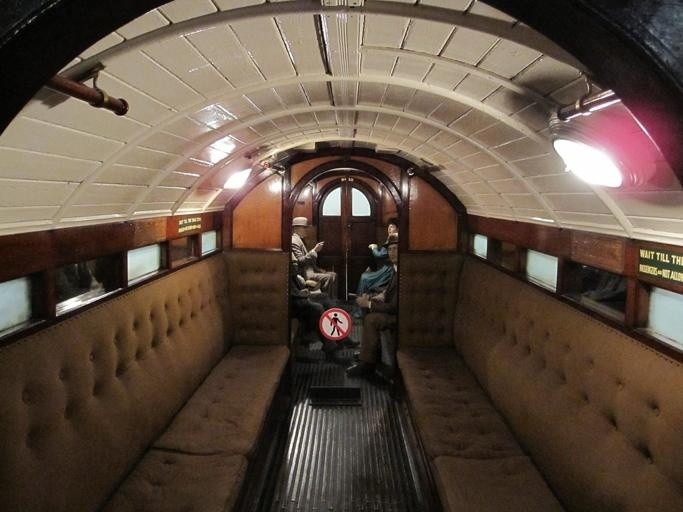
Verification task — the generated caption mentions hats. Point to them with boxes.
[380,232,399,249]
[291,216,312,227]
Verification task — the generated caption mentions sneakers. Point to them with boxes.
[354,310,362,318]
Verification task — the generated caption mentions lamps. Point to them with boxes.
[548,113,657,188]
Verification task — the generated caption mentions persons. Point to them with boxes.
[290,216,338,305]
[351,217,399,321]
[345,232,398,379]
[289,259,360,364]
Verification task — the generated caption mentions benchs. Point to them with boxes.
[396,250,683,512]
[0,247,294,512]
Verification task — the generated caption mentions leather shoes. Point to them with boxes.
[345,338,359,348]
[352,351,361,358]
[345,361,365,377]
[325,352,350,365]
[336,302,345,308]
